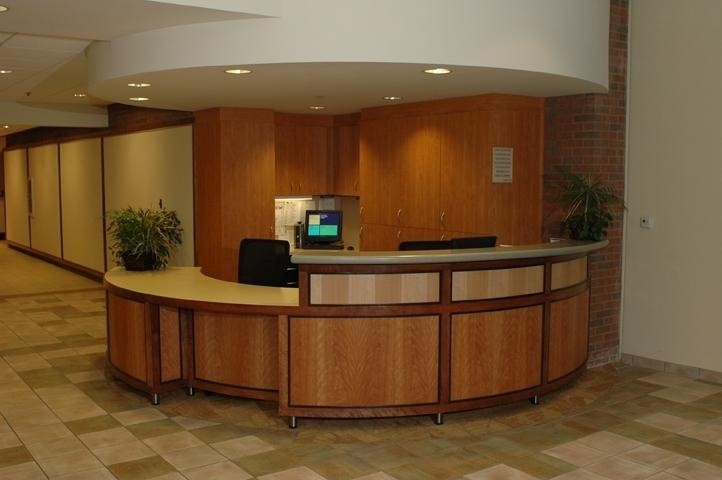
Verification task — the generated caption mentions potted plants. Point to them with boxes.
[551,166,619,244]
[106,204,181,272]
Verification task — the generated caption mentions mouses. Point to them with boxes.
[348,246,353,250]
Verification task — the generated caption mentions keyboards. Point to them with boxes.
[304,245,343,250]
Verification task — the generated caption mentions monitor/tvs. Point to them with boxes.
[452,235,496,248]
[305,209,343,242]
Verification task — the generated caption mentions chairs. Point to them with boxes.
[236,235,297,287]
